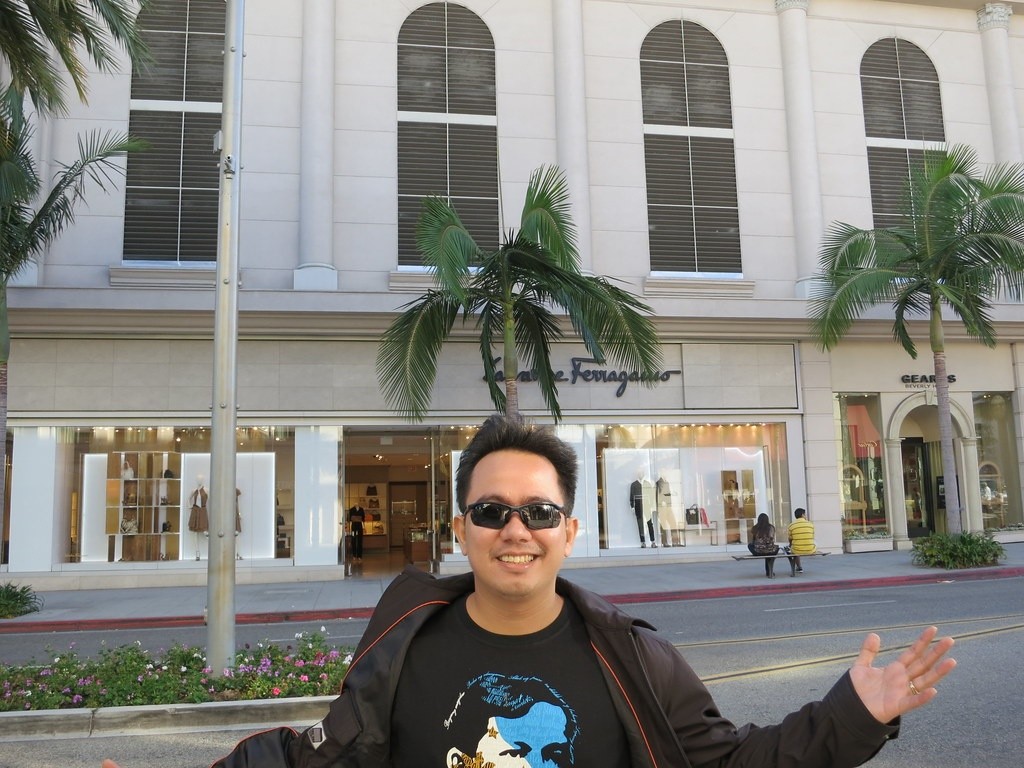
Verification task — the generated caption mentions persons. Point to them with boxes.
[187,473,209,561]
[347,502,366,560]
[101,412,954,768]
[981,481,993,532]
[747,513,779,578]
[788,508,817,573]
[235,488,244,561]
[630,467,685,548]
[911,487,921,519]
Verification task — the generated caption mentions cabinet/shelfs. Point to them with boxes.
[107,450,182,562]
[342,464,391,553]
[403,527,431,565]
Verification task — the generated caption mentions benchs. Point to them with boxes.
[731,551,832,579]
[678,520,718,547]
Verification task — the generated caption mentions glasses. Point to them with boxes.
[462,500,568,530]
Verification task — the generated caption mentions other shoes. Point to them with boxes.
[795,567,804,573]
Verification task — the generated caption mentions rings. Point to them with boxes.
[909,680,919,695]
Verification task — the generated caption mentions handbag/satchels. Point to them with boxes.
[121,518,138,533]
[369,498,379,508]
[359,497,367,509]
[686,504,698,524]
[373,522,384,535]
[364,510,373,522]
[277,514,285,526]
[124,461,135,478]
[371,509,380,521]
[366,483,378,495]
